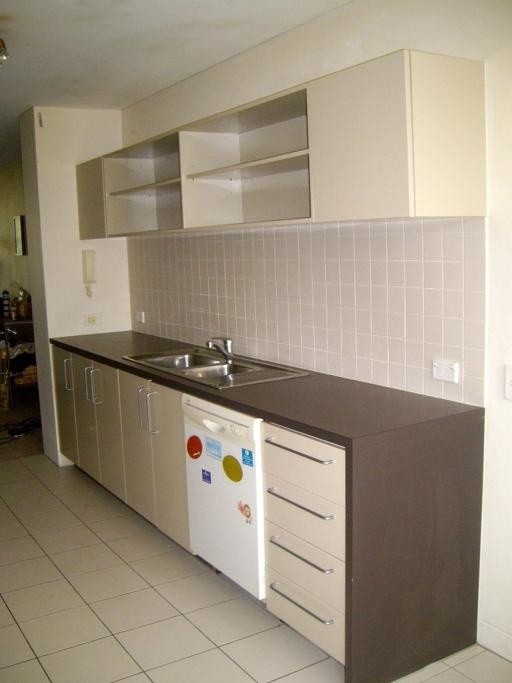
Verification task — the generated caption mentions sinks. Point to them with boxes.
[121,348,221,369]
[181,364,264,390]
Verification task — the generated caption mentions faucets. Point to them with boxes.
[206,337,234,363]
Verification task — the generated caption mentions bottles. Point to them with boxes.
[4,283,26,321]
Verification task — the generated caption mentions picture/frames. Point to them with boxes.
[12,216,26,257]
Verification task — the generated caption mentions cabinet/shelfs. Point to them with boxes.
[2,320,38,409]
[72,353,189,556]
[51,345,78,468]
[74,49,486,245]
[263,418,484,683]
